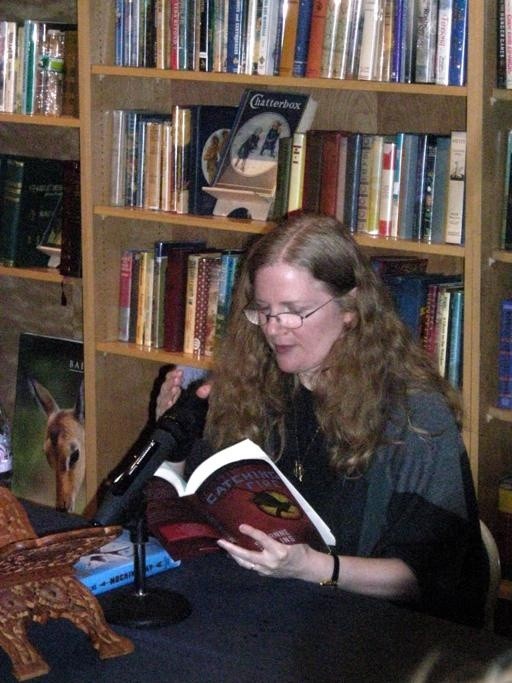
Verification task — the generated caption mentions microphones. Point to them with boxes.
[89,404,198,528]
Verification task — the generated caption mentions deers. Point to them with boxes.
[25,373,86,517]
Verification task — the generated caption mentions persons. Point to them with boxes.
[152,209,490,636]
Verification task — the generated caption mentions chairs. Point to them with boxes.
[479,520,501,634]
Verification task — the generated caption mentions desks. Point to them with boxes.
[0,497,512,683]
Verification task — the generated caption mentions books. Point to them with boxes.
[0,18,83,278]
[369,255,466,391]
[109,87,469,243]
[114,0,466,88]
[491,0,511,547]
[152,438,337,563]
[116,239,247,358]
[11,333,88,515]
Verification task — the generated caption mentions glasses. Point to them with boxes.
[242,291,342,330]
